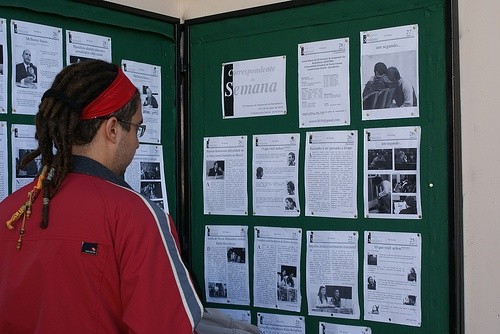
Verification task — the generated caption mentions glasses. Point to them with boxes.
[104,116,145,137]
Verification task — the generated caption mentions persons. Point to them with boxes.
[256,167,265,179]
[144,88,159,108]
[229,251,242,262]
[369,150,417,214]
[315,286,342,310]
[1,58,206,334]
[16,51,37,86]
[287,151,296,167]
[330,74,342,105]
[408,295,417,305]
[363,63,417,109]
[277,270,296,302]
[371,305,379,314]
[367,253,374,265]
[285,181,296,211]
[408,267,417,282]
[141,165,163,212]
[368,277,376,290]
[207,163,224,177]
